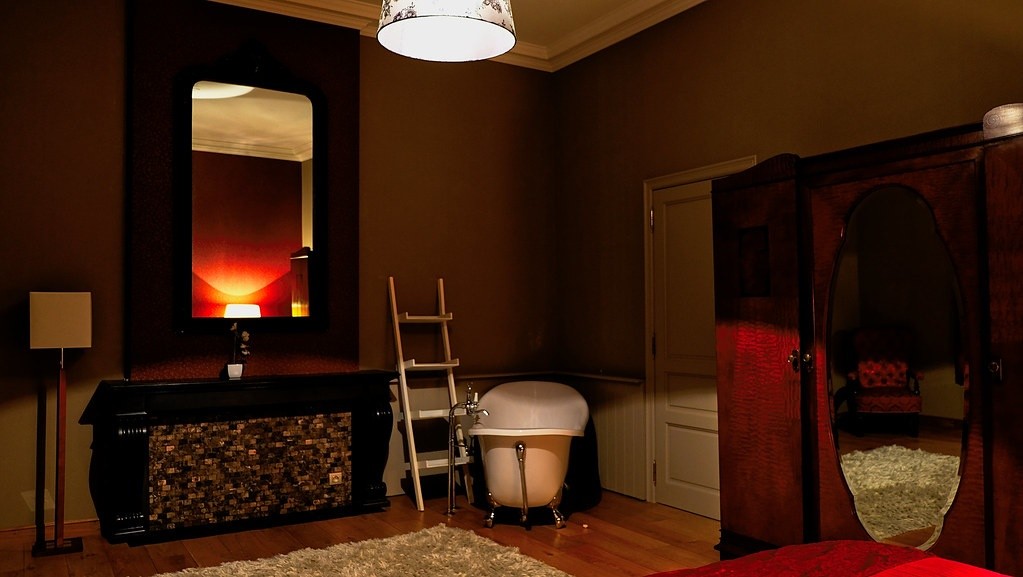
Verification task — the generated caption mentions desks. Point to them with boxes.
[88,370,398,550]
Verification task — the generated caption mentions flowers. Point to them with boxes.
[229,322,251,365]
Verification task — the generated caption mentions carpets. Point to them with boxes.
[157,522,575,577]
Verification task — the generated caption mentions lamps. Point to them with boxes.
[29,290,92,556]
[377,1,516,61]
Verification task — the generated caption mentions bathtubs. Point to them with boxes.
[466,380,591,509]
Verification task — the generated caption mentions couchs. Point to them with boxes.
[844,354,924,438]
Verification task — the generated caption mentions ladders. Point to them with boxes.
[388,276,474,512]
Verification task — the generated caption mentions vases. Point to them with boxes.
[227,364,244,382]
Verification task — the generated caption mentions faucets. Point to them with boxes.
[463,379,491,419]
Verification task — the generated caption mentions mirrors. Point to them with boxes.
[179,74,331,335]
[822,180,975,552]
